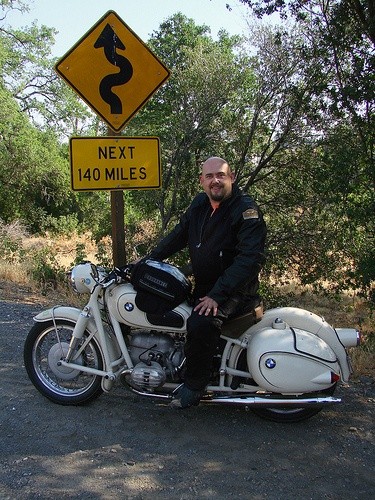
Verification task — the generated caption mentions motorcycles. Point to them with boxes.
[24,243,362,424]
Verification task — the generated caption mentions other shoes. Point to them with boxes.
[171,382,205,409]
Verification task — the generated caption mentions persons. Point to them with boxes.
[129,158,267,407]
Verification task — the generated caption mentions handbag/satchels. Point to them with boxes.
[130,257,193,305]
